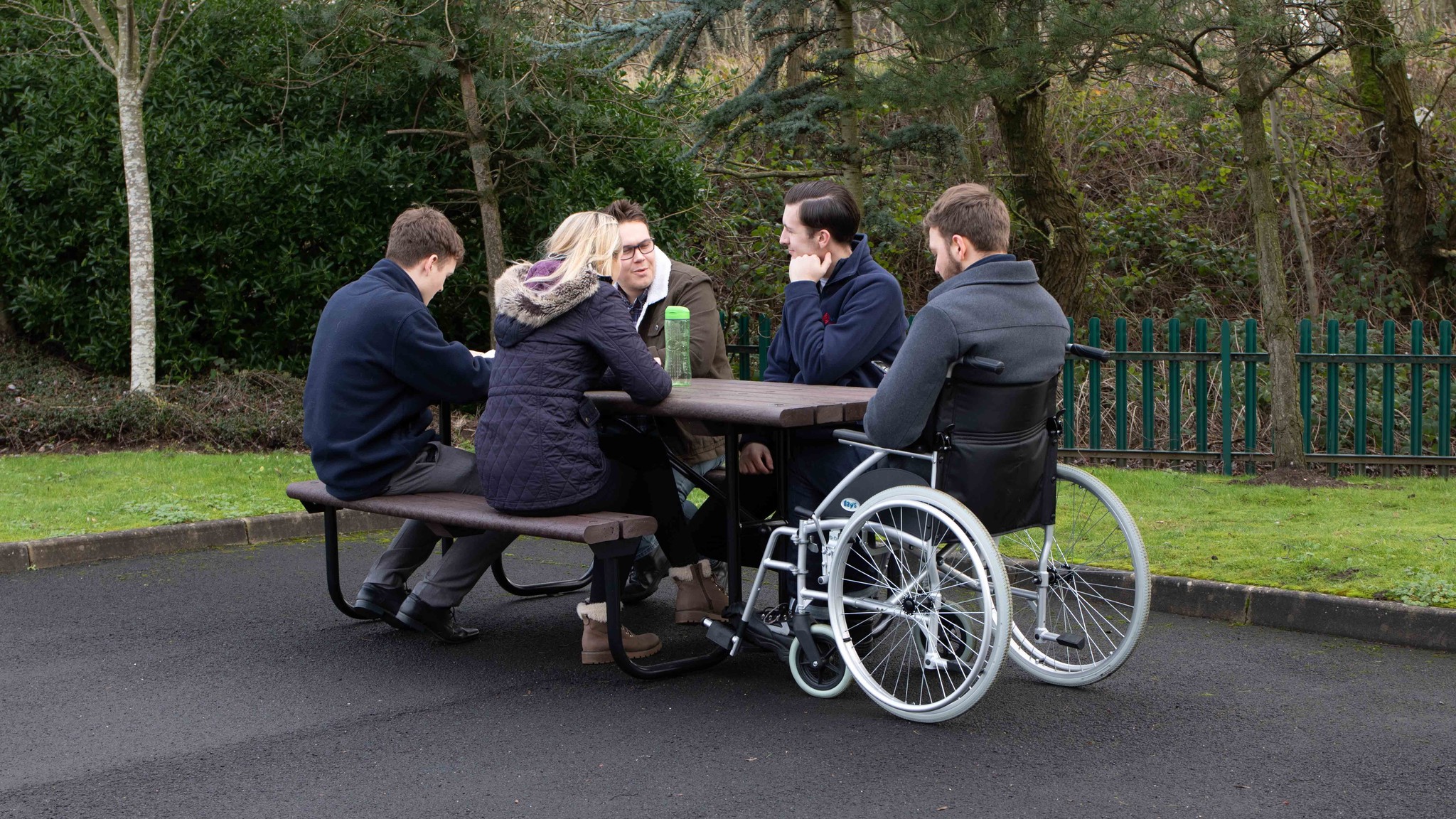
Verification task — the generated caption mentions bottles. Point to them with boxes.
[664,304,691,387]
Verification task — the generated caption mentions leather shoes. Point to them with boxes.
[395,592,479,645]
[353,581,415,631]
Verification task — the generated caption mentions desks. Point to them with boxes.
[436,375,882,615]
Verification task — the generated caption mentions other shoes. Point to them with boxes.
[710,560,728,592]
[621,544,672,605]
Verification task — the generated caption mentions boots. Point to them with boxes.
[576,597,662,663]
[668,559,729,624]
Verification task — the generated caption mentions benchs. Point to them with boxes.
[281,477,729,688]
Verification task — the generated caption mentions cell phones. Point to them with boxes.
[871,360,890,374]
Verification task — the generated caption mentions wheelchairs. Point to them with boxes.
[702,343,1154,724]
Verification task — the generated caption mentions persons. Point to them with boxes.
[599,195,742,605]
[474,211,750,664]
[688,183,1070,670]
[302,207,523,639]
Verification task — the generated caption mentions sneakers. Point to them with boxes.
[815,618,873,657]
[728,600,808,665]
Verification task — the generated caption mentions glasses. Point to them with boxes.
[620,236,656,260]
